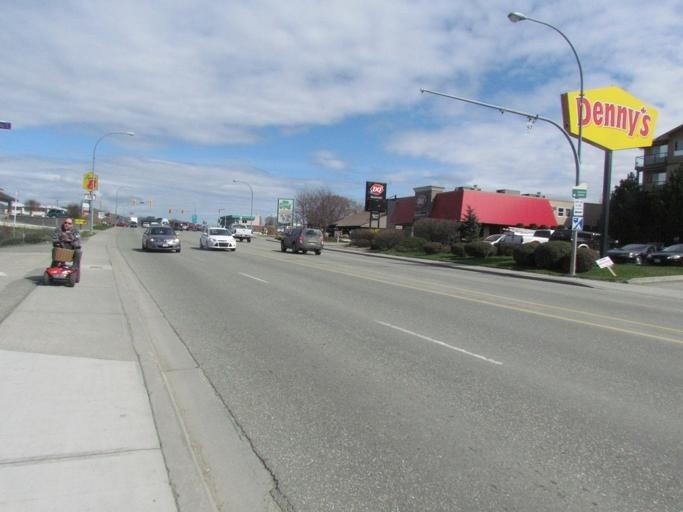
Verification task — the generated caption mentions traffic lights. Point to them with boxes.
[169,208,172,214]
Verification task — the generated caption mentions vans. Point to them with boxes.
[281,228,323,255]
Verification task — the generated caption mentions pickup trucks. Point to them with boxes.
[228,224,252,242]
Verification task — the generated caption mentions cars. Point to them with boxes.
[482,227,683,266]
[142,219,208,232]
[200,227,236,250]
[48,209,66,218]
[141,224,180,252]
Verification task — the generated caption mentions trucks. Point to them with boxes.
[130,216,138,227]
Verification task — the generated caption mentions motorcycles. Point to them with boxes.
[44,233,81,287]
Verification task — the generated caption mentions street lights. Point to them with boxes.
[89,131,134,233]
[234,179,253,223]
[508,11,585,273]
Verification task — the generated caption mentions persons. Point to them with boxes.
[52,216,83,270]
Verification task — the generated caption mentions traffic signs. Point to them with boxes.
[573,199,584,217]
[573,188,586,197]
